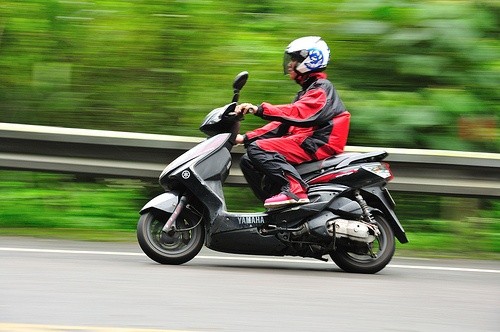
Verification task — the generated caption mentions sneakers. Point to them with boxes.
[264,192,310,208]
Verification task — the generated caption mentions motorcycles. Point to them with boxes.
[137,72,408,274]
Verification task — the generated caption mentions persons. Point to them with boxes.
[233,34,352,211]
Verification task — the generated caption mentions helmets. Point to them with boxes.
[286,36,331,75]
